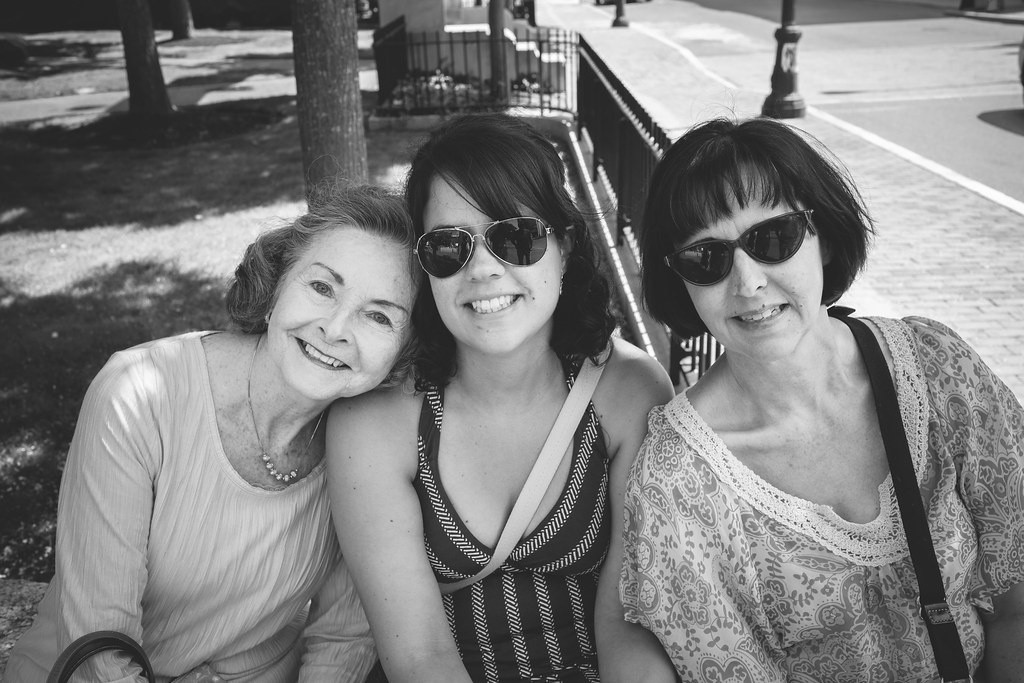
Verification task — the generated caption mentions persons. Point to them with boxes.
[326,113,675,683]
[618,119,1024,683]
[5,186,420,683]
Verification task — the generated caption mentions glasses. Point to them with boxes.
[663,209,820,286]
[412,214,577,279]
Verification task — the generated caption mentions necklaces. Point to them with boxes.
[246,328,328,484]
